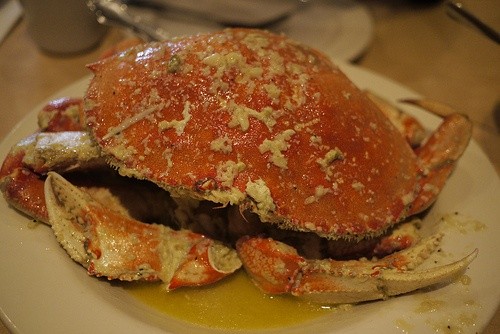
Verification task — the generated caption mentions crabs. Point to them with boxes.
[0,28,480,305]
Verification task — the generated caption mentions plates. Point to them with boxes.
[124,0,374,64]
[0,55,499,334]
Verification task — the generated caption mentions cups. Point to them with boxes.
[19,0,108,58]
